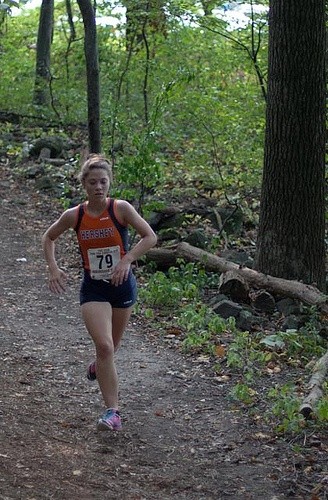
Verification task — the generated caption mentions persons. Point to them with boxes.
[39,154,160,431]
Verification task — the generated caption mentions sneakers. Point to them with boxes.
[87,361,98,380]
[97,408,124,433]
